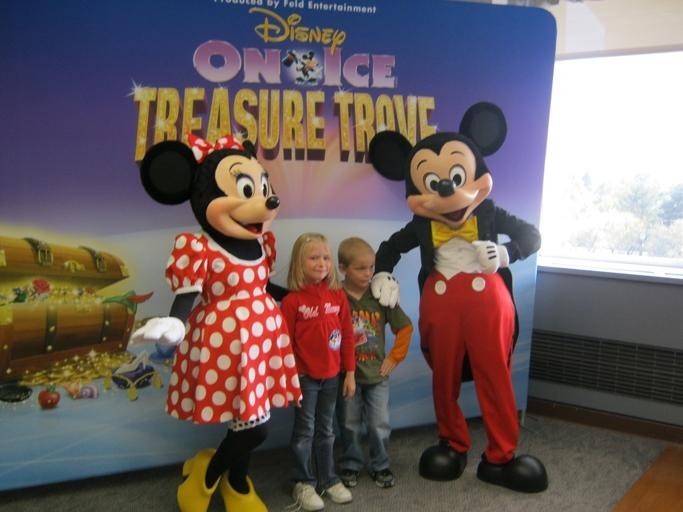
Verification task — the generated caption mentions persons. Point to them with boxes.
[280,233,361,510]
[334,236,414,490]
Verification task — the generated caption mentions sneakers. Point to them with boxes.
[284,481,325,512]
[327,469,359,503]
[369,469,393,487]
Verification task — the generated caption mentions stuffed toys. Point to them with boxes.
[125,129,294,511]
[366,102,548,493]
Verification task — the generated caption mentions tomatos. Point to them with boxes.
[38,383,60,408]
